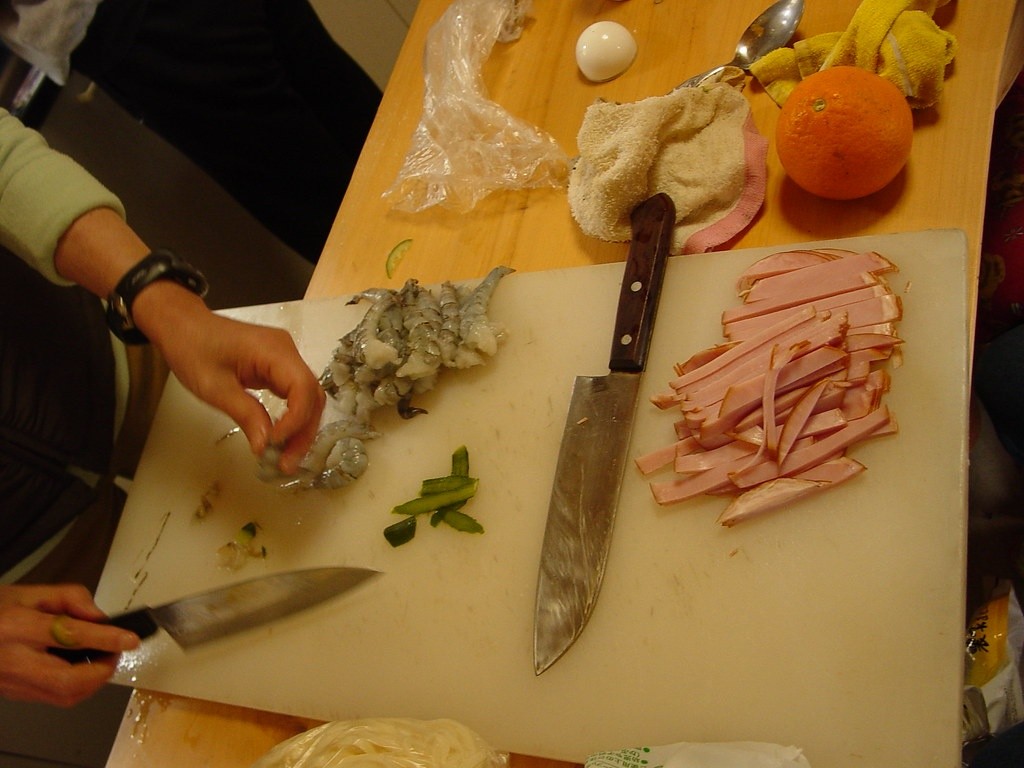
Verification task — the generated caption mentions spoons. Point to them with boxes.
[565,0,802,174]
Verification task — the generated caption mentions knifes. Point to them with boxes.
[46,568,381,665]
[532,193,676,675]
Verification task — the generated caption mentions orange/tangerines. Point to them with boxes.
[775,65,913,200]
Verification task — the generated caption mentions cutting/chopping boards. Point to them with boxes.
[93,230,966,767]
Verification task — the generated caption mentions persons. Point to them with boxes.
[0,105,327,706]
[1,0,386,264]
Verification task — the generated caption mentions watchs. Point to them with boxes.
[105,241,209,346]
